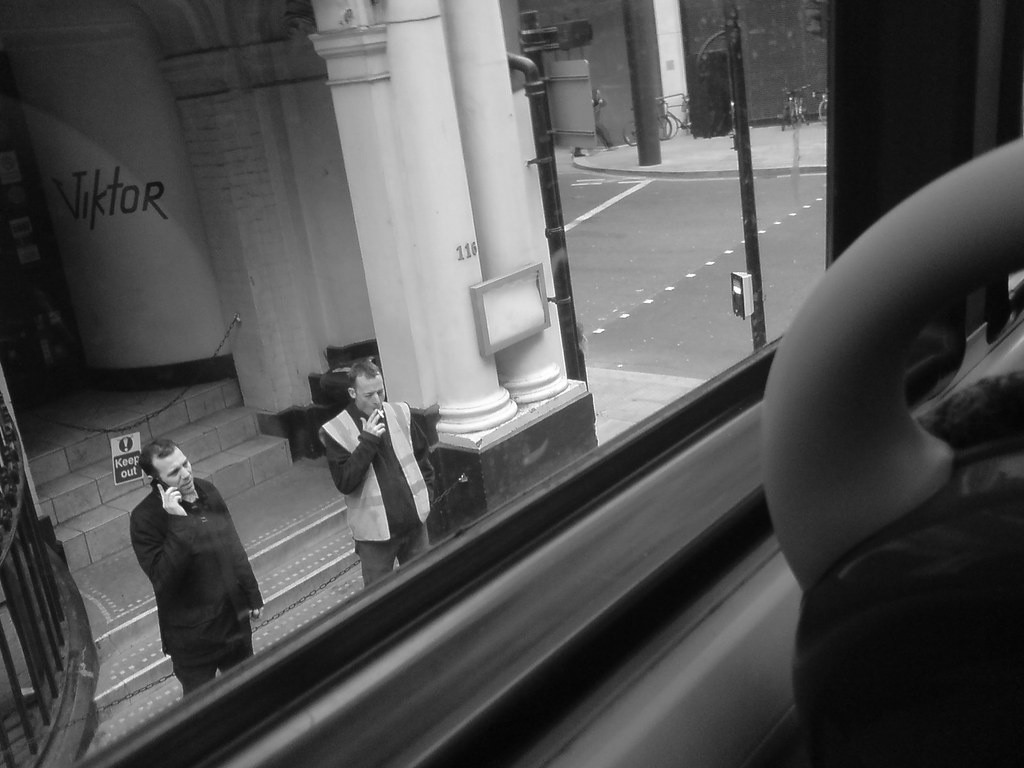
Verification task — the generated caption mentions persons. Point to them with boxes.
[130,438,264,698]
[319,361,436,589]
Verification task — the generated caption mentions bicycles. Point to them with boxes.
[623,99,672,147]
[778,84,812,132]
[812,90,828,126]
[657,94,692,140]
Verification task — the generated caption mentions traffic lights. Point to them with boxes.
[730,272,755,321]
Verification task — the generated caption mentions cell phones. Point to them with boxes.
[150,477,171,492]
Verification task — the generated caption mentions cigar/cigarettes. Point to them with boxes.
[377,408,384,419]
